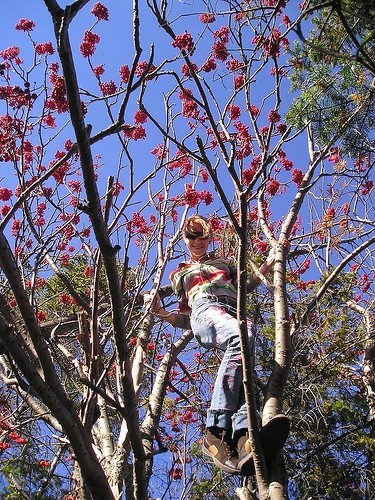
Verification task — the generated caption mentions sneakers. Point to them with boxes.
[238,414,290,476]
[202,430,242,474]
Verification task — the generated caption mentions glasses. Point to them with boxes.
[186,231,209,240]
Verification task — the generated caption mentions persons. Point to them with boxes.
[144,217,291,476]
